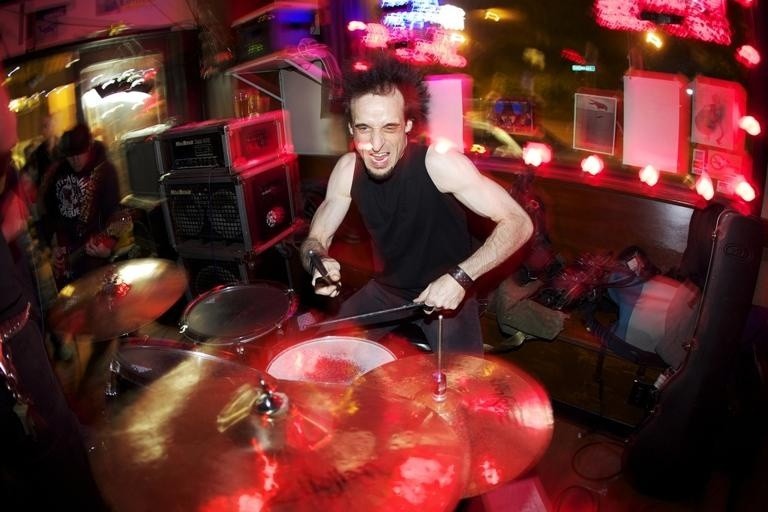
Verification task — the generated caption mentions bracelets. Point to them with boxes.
[446,262,476,291]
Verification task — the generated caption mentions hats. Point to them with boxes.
[62,129,89,157]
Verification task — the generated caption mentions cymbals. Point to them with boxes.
[90,376,465,512]
[354,354,554,500]
[51,257,190,343]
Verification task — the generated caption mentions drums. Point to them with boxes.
[105,338,278,409]
[266,335,397,384]
[180,282,298,371]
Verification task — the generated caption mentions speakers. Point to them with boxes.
[175,243,294,302]
[154,112,287,175]
[160,155,296,263]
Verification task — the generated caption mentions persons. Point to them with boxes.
[31,122,126,389]
[0,76,80,472]
[298,56,537,364]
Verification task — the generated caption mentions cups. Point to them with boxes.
[234,85,271,123]
